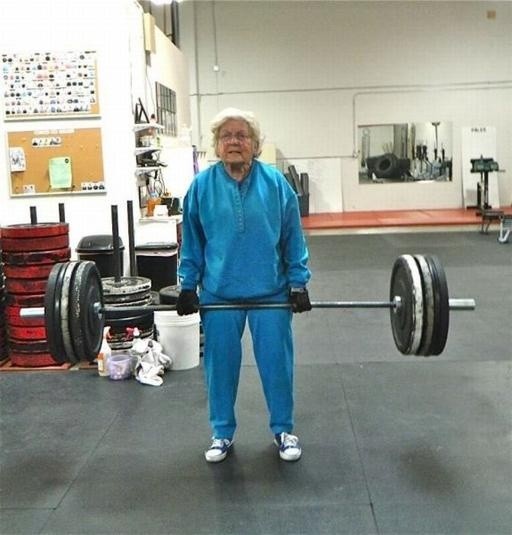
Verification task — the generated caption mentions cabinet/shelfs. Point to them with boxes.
[134,123,165,177]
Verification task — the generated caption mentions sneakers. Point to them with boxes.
[205,436,235,463]
[273,431,302,462]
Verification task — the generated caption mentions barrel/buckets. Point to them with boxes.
[152,311,201,371]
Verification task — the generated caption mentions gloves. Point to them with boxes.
[288,288,312,313]
[177,290,201,316]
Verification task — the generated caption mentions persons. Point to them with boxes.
[173,110,314,465]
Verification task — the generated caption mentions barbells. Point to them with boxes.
[20,254,475,363]
